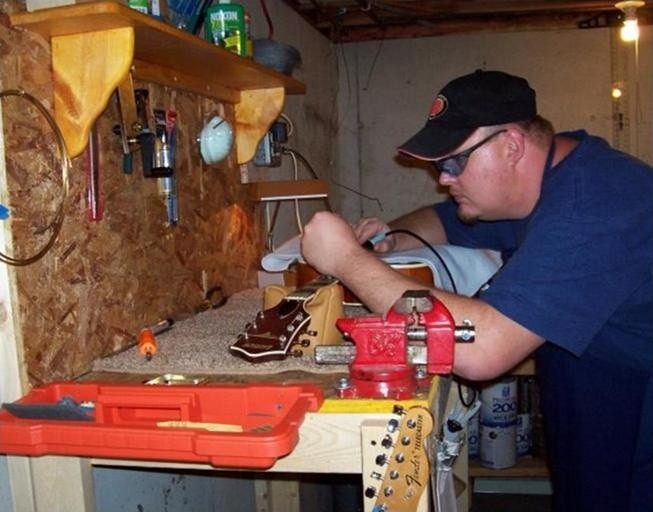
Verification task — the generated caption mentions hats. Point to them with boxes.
[396,68,535,161]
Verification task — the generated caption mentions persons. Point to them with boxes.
[299,67,652,510]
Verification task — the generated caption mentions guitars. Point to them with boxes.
[228,262,435,362]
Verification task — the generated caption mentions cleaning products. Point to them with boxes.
[205,0,247,58]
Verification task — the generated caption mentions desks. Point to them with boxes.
[3,284,469,511]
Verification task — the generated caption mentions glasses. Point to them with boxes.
[434,130,509,176]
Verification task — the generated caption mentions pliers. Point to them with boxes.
[194,287,228,313]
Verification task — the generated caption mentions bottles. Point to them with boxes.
[207,0,253,58]
